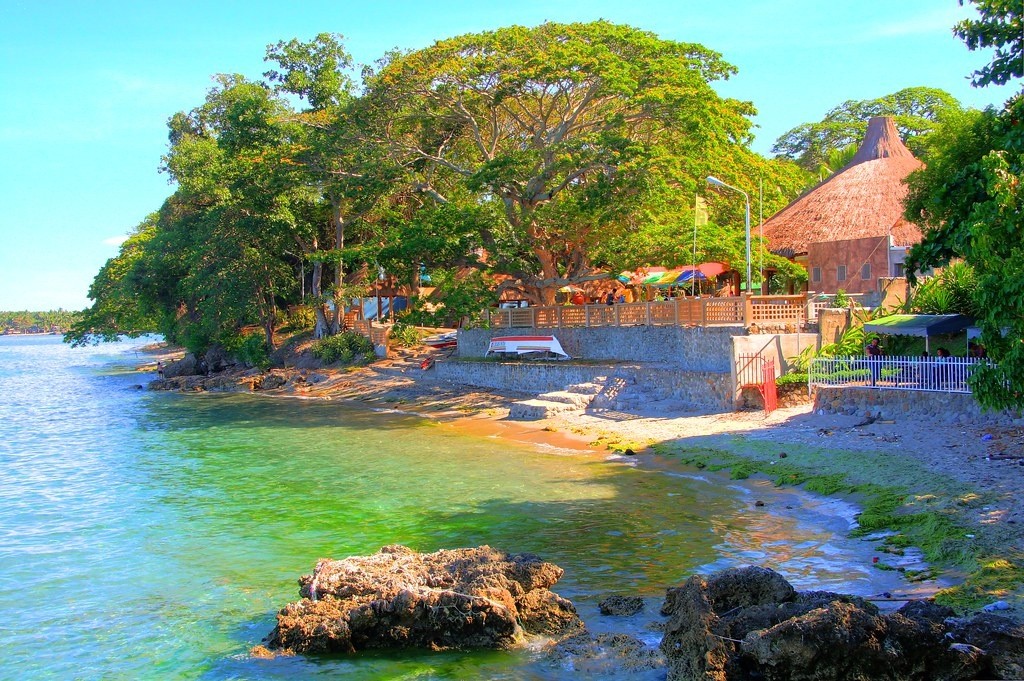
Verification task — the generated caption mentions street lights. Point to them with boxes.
[706,175,753,294]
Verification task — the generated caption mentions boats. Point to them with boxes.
[422,331,458,350]
[484,335,569,362]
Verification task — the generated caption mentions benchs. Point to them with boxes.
[484,335,571,363]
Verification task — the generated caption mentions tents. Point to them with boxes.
[964,324,1014,359]
[861,313,973,358]
[624,268,708,297]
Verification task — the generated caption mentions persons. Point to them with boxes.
[866,337,882,386]
[641,287,664,302]
[157,362,165,379]
[966,341,986,359]
[729,290,735,297]
[913,347,948,381]
[171,359,175,364]
[601,288,625,305]
[849,297,855,308]
[573,291,584,306]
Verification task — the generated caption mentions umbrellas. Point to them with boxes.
[557,285,586,303]
